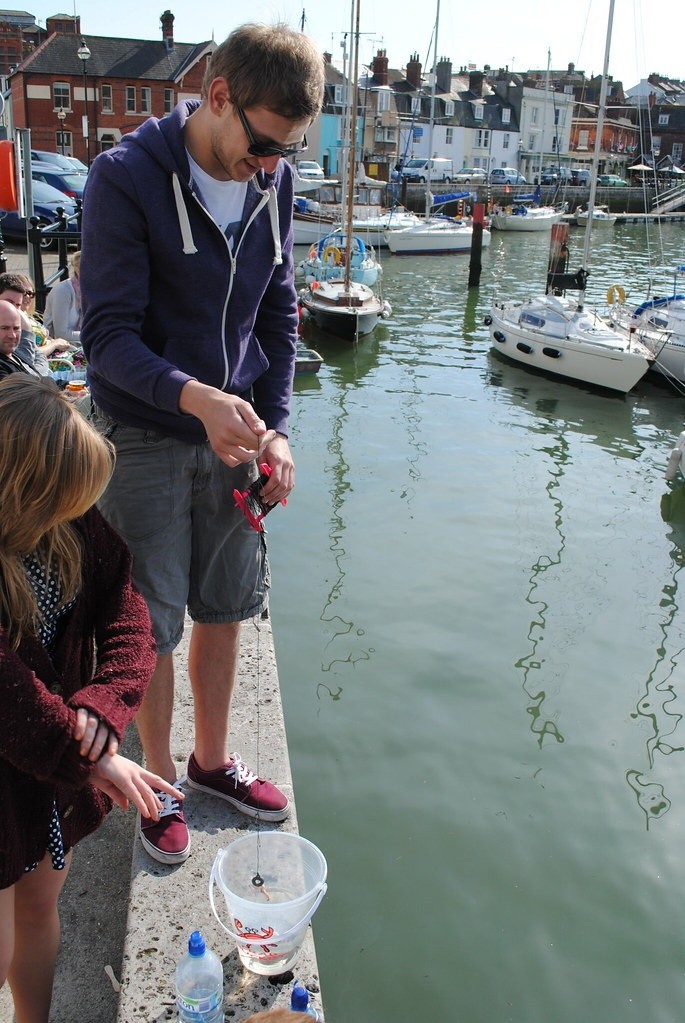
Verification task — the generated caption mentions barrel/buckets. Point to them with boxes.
[208,830,328,977]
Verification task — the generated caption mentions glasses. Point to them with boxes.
[23,290,36,298]
[230,86,309,156]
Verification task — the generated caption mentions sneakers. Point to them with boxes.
[140,775,191,864]
[186,751,291,822]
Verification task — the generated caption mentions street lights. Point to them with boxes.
[77,35,94,167]
[57,106,67,155]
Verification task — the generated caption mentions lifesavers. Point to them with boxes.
[505,207,512,215]
[324,247,340,263]
[607,284,626,304]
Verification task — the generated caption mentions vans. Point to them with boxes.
[400,159,452,184]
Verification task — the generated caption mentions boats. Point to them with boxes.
[301,229,383,288]
[294,349,324,373]
[489,293,656,399]
[489,203,570,232]
[604,293,685,387]
[300,278,384,343]
[330,213,424,250]
[289,177,402,246]
[575,207,619,229]
[381,220,491,253]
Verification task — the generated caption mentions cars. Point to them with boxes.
[296,160,325,180]
[598,174,628,187]
[0,146,90,208]
[453,167,487,185]
[534,166,573,186]
[570,169,601,187]
[489,168,526,186]
[0,176,85,250]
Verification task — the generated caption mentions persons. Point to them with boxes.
[79,16,323,865]
[0,241,155,1023]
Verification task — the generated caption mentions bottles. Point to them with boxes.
[287,978,322,1023]
[173,930,226,1023]
